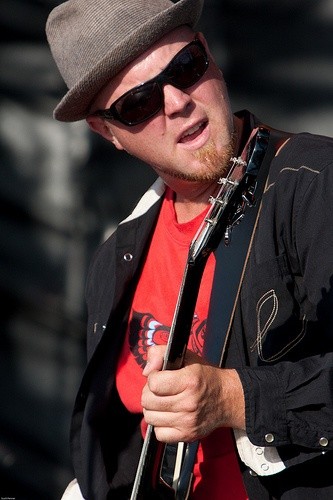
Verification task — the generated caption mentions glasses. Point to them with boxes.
[94,35,210,128]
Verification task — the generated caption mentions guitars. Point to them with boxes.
[129,124,270,500]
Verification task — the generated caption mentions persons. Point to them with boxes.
[44,0,333,500]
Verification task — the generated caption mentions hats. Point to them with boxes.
[44,1,215,122]
